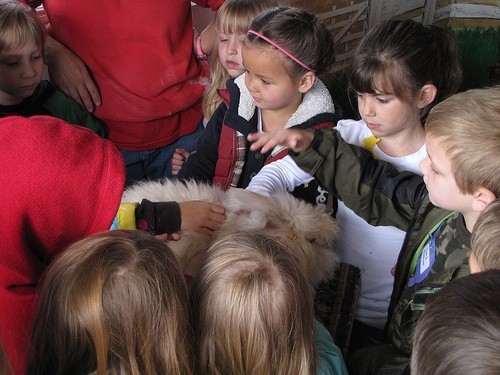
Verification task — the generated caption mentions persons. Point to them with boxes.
[193,228,350,375]
[0,0,500,375]
[27,228,198,375]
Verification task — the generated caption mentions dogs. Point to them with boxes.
[116,174,339,298]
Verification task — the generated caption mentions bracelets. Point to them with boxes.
[195,36,208,60]
